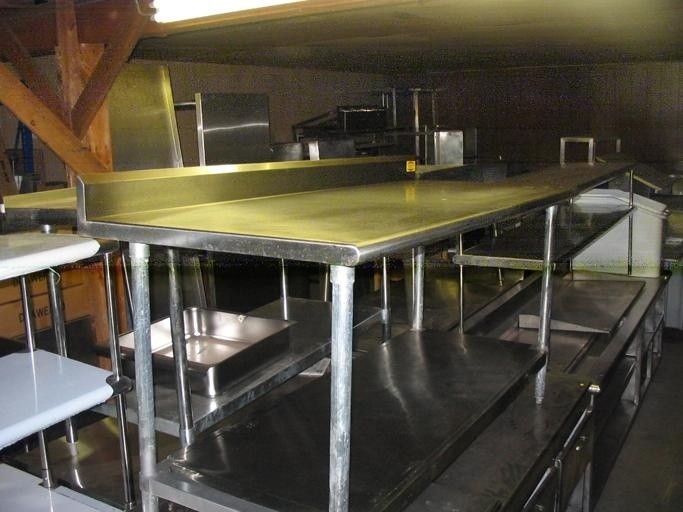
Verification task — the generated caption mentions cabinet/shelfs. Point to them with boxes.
[0,85,669,512]
